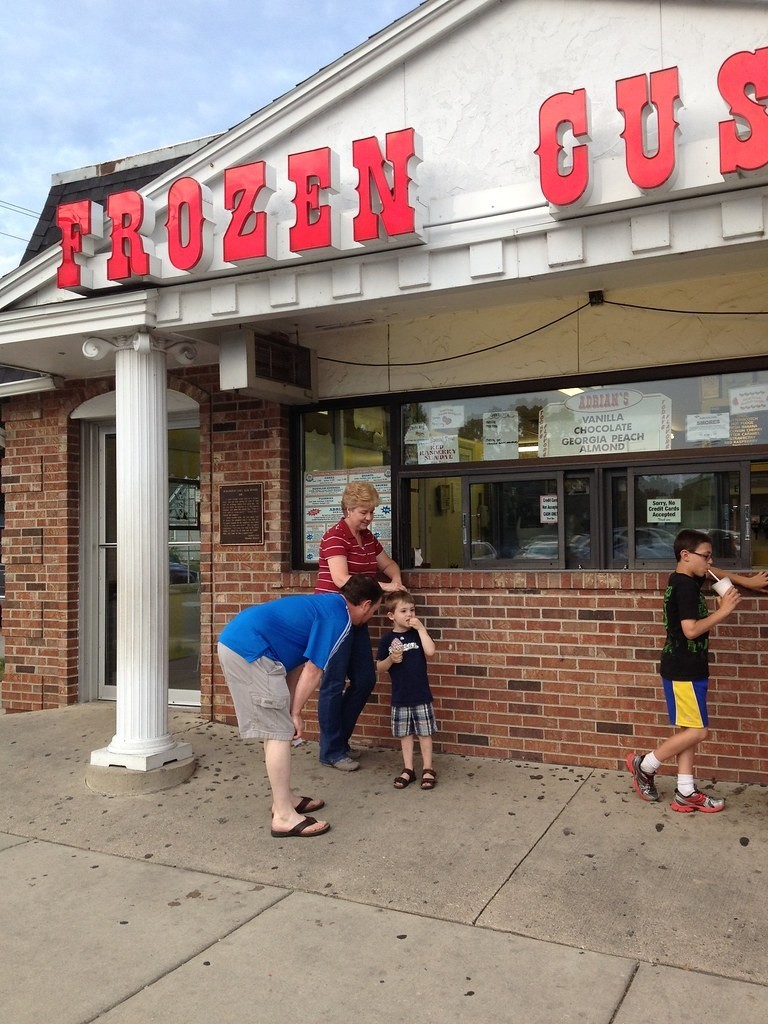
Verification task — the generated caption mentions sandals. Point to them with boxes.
[420,768,437,790]
[393,769,416,789]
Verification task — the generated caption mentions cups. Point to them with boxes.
[712,576,739,601]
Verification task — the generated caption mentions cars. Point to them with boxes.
[169,561,197,584]
[471,541,497,561]
[513,527,751,562]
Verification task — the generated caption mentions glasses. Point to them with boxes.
[687,549,713,562]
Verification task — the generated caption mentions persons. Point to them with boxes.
[626,530,768,812]
[218,481,437,837]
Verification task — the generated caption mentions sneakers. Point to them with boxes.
[627,752,660,800]
[345,748,362,757]
[672,787,726,813]
[322,757,360,771]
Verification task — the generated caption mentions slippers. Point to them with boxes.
[271,815,331,837]
[271,796,324,818]
